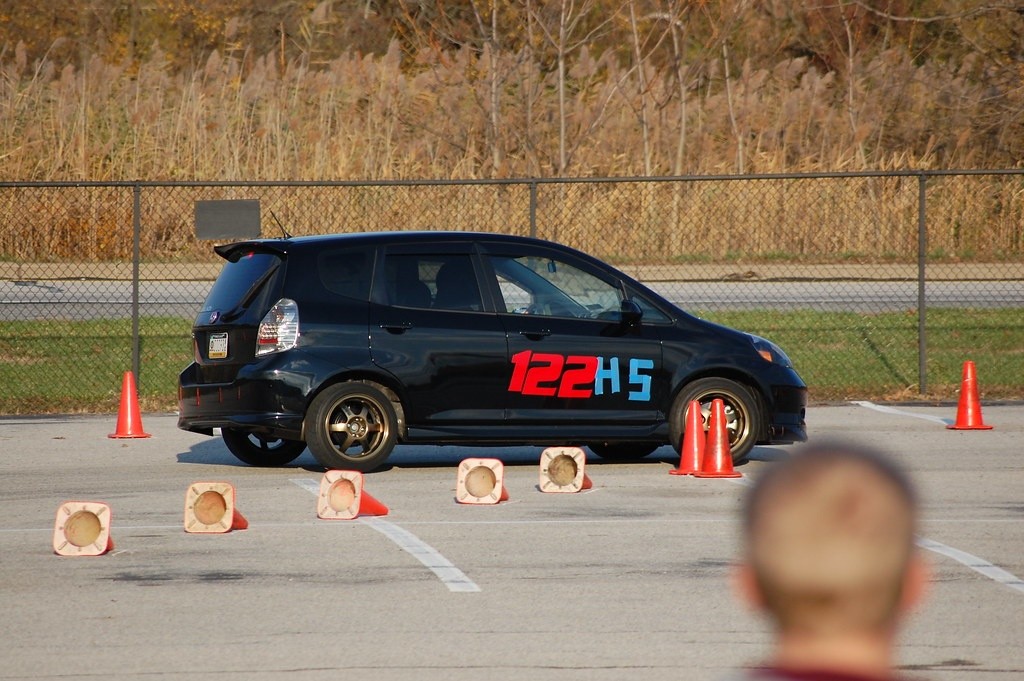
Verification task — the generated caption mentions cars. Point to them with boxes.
[177,230,809,474]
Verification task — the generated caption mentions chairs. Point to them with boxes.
[385,256,431,308]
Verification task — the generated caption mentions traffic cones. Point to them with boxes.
[317,469,389,519]
[179,386,186,422]
[236,385,241,402]
[669,399,706,476]
[52,500,114,556]
[217,387,223,405]
[692,398,743,479]
[183,482,249,533]
[455,456,509,505]
[946,359,994,430]
[538,446,592,493]
[195,387,201,408]
[108,371,152,438]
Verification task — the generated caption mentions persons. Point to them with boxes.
[741,446,923,681]
